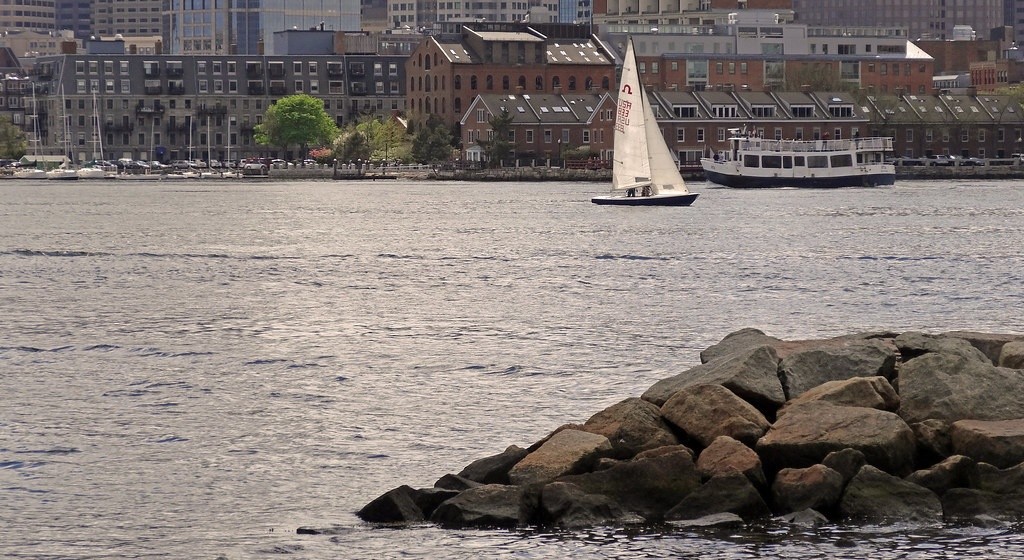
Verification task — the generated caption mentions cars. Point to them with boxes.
[893,155,922,166]
[1010,153,1024,166]
[84,156,317,171]
[956,156,974,166]
[965,157,984,166]
[918,155,949,166]
[946,154,963,166]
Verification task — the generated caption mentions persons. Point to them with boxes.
[626,188,638,197]
[713,151,724,164]
[821,131,830,151]
[854,132,860,150]
[641,185,652,197]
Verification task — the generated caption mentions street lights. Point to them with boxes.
[384,137,388,166]
[342,141,346,163]
[557,138,562,166]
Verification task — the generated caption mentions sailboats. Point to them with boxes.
[77,88,107,179]
[182,115,201,179]
[45,84,79,179]
[201,117,223,179]
[592,33,701,207]
[12,83,47,179]
[221,116,243,179]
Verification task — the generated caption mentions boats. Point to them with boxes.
[165,171,187,179]
[115,167,162,180]
[700,130,897,188]
